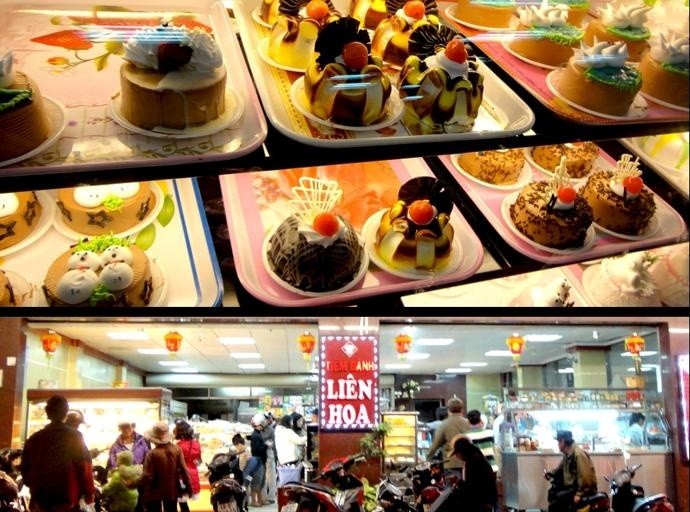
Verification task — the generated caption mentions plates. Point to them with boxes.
[437,136,687,266]
[522,143,592,185]
[445,3,522,32]
[500,32,570,68]
[1,0,270,176]
[248,0,272,29]
[379,60,410,71]
[285,76,409,134]
[0,175,228,309]
[255,222,375,300]
[141,248,169,307]
[436,1,690,125]
[105,82,246,141]
[639,88,689,115]
[615,138,690,201]
[450,146,535,191]
[581,264,662,307]
[400,264,592,307]
[545,71,646,125]
[357,204,468,282]
[218,156,484,307]
[52,181,172,244]
[498,193,602,259]
[628,138,690,176]
[226,0,536,151]
[591,211,665,243]
[0,190,56,262]
[254,33,307,76]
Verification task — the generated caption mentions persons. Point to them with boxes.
[232,433,253,512]
[625,412,651,446]
[123,422,192,512]
[492,400,504,473]
[95,450,144,512]
[509,390,516,400]
[105,419,152,512]
[448,434,499,512]
[416,407,450,473]
[251,417,271,506]
[290,412,309,464]
[260,414,278,503]
[275,415,307,467]
[544,430,598,512]
[21,396,97,512]
[0,449,24,491]
[173,419,202,512]
[65,409,100,512]
[428,398,474,512]
[462,410,502,512]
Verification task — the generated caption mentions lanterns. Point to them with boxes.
[299,333,314,361]
[505,335,526,359]
[43,334,61,359]
[395,335,412,357]
[167,332,182,351]
[626,333,645,356]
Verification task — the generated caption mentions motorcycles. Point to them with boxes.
[603,451,675,512]
[283,452,367,512]
[203,447,249,512]
[376,458,450,512]
[543,465,610,512]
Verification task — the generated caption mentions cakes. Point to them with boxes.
[641,133,689,171]
[0,52,48,161]
[122,28,227,130]
[454,0,511,27]
[511,155,593,249]
[260,1,280,23]
[373,0,442,68]
[374,177,455,273]
[0,189,42,249]
[585,3,651,63]
[305,17,391,127]
[42,235,152,307]
[268,177,363,291]
[564,3,589,30]
[561,40,645,115]
[60,181,155,235]
[580,154,655,234]
[531,143,599,178]
[268,0,343,68]
[396,24,485,128]
[635,30,690,111]
[0,272,17,307]
[586,251,660,306]
[351,1,388,30]
[459,148,524,184]
[509,2,583,67]
[649,244,690,306]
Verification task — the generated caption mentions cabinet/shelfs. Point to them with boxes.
[500,403,674,511]
[380,410,420,472]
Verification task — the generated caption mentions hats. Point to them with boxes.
[146,422,174,445]
[448,436,471,456]
[553,431,572,443]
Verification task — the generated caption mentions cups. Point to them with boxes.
[0,92,71,166]
